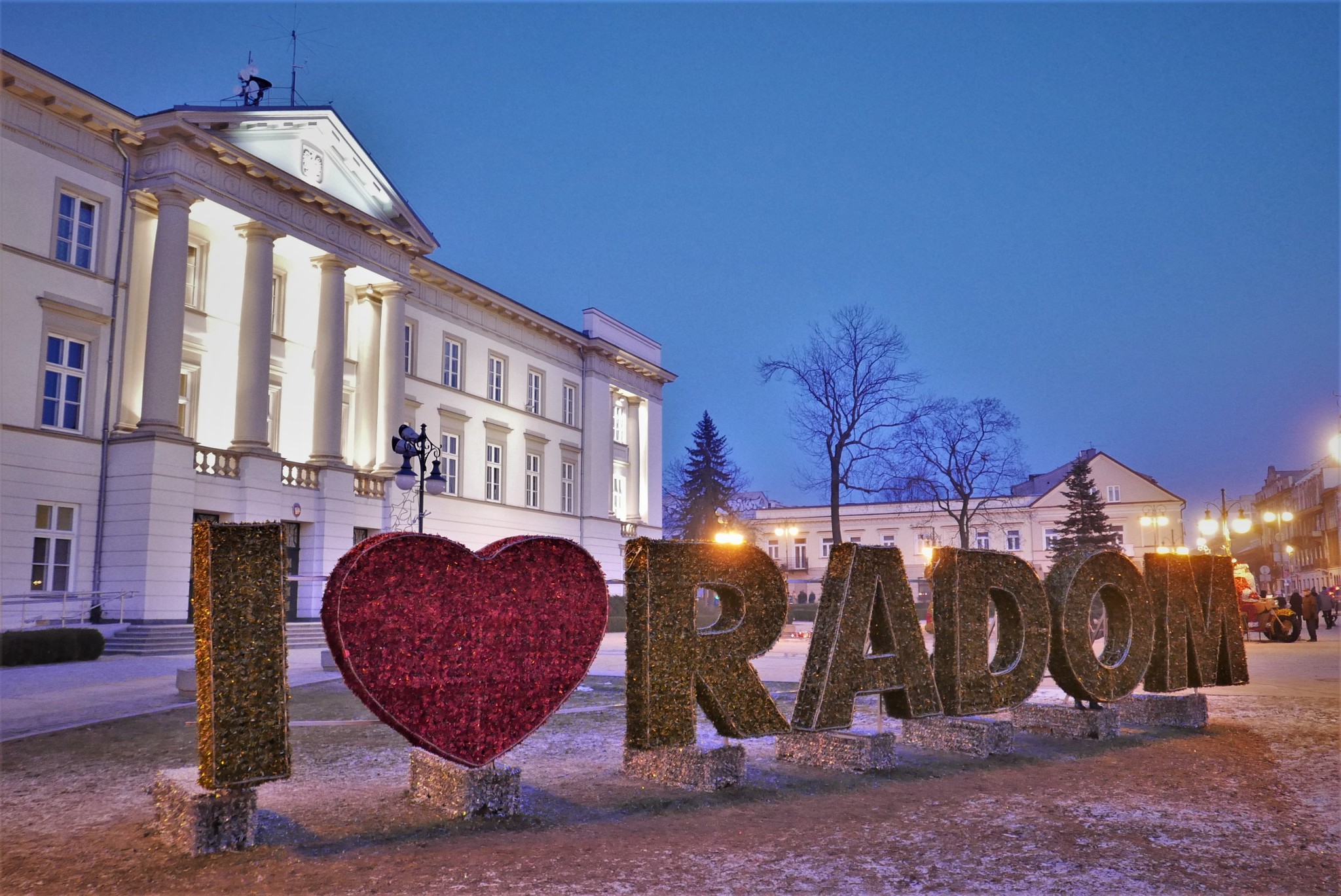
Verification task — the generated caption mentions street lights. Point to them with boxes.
[1159,529,1188,557]
[922,527,943,561]
[394,422,447,535]
[1199,488,1251,557]
[1264,497,1293,579]
[1141,504,1170,552]
[775,516,799,593]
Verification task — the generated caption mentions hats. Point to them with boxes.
[1303,588,1309,591]
[1293,589,1299,593]
[1311,586,1316,593]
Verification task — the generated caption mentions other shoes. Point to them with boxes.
[1307,638,1317,642]
[1325,626,1331,630]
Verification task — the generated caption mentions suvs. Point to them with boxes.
[1329,584,1341,607]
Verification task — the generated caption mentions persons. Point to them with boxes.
[809,591,816,603]
[1274,591,1287,609]
[792,590,799,604]
[798,590,808,603]
[1289,589,1303,620]
[1311,586,1319,630]
[1259,590,1267,599]
[1302,588,1319,642]
[1318,587,1335,629]
